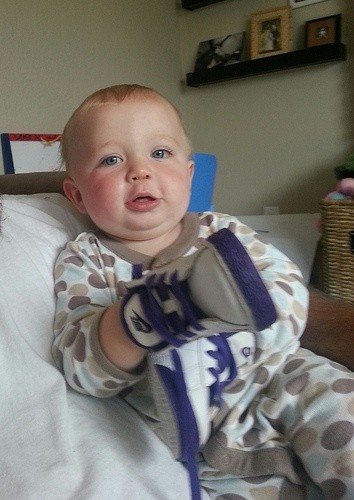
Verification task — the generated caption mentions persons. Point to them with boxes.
[50,84,354,500]
[263,25,280,53]
[317,28,328,39]
[207,45,236,69]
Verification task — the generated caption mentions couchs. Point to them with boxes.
[1,171,354,500]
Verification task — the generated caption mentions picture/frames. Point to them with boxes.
[305,13,342,49]
[249,5,293,61]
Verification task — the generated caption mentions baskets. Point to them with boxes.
[319,199,354,304]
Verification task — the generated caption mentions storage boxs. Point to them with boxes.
[320,199,354,304]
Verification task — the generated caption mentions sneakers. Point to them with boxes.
[120,228,278,349]
[146,332,261,500]
